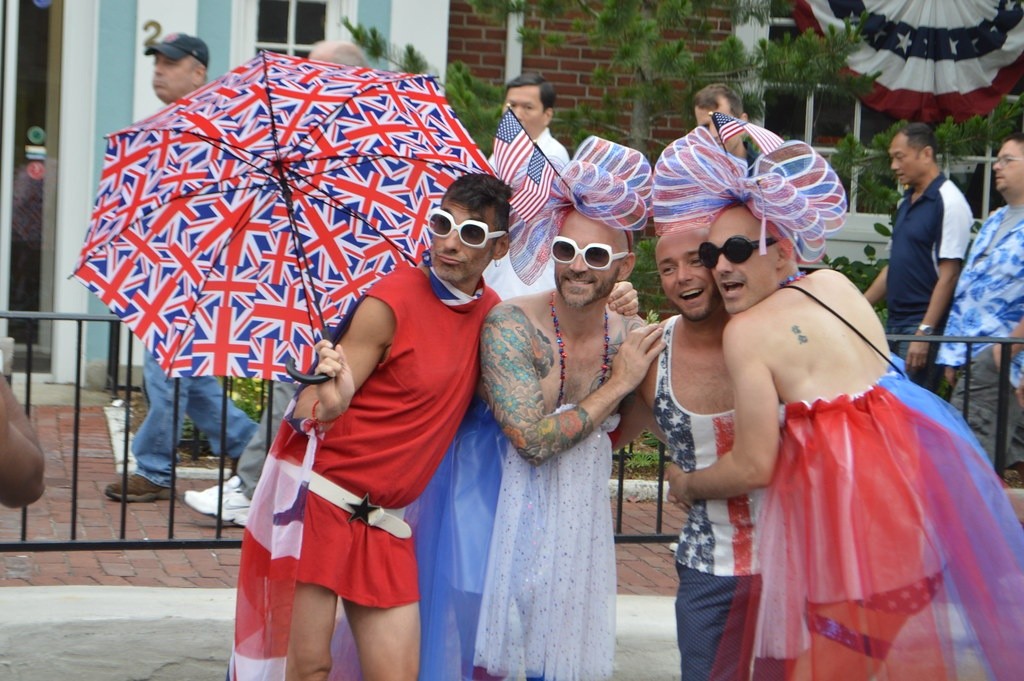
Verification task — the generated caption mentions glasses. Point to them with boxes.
[998,155,1022,167]
[551,237,628,272]
[696,234,777,269]
[427,206,504,249]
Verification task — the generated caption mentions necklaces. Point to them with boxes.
[549,290,609,408]
[779,270,806,288]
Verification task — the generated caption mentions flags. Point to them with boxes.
[713,112,785,156]
[493,108,558,223]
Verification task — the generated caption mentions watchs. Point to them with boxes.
[918,322,935,335]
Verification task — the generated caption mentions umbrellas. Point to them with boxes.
[67,48,497,386]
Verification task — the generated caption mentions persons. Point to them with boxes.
[405,137,669,681]
[607,84,1024,681]
[104,32,260,499]
[184,40,367,527]
[0,352,48,508]
[482,73,570,306]
[226,172,640,681]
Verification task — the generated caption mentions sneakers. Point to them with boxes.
[104,473,178,502]
[185,476,250,528]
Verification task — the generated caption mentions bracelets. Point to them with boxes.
[313,400,343,425]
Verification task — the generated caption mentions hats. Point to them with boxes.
[144,33,209,67]
[508,135,652,285]
[645,125,737,233]
[728,139,852,265]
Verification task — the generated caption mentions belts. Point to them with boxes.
[283,456,413,539]
